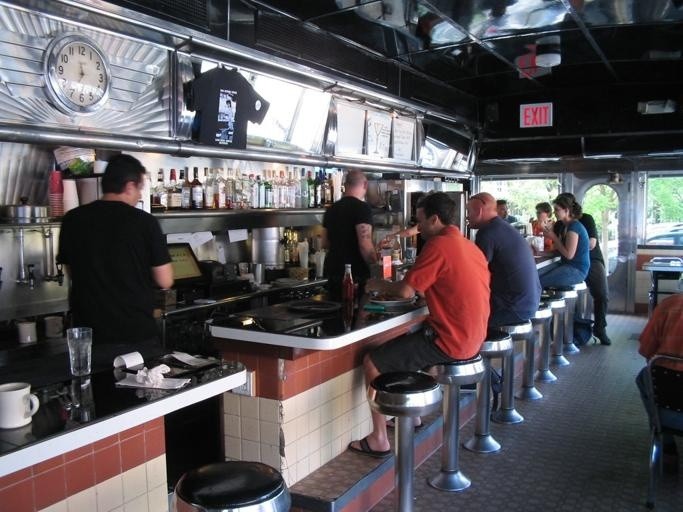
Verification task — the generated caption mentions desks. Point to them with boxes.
[642,262,683,320]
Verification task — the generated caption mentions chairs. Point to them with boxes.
[636,354,683,510]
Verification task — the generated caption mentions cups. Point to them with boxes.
[65,326,92,376]
[530,236,543,252]
[239,263,248,277]
[1,424,36,455]
[0,382,39,429]
[46,170,79,218]
[44,315,63,338]
[67,379,97,426]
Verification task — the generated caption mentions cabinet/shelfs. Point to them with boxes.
[0,123,385,237]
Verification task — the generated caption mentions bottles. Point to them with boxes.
[146,167,344,213]
[342,264,354,300]
[392,233,401,262]
[17,321,37,343]
[283,225,298,264]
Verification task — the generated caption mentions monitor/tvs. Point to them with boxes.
[166,243,203,280]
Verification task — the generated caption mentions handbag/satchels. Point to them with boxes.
[570,314,597,349]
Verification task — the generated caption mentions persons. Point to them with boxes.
[531,202,556,245]
[558,192,612,345]
[348,193,491,459]
[379,224,420,249]
[496,199,517,224]
[634,293,683,473]
[320,170,377,279]
[539,195,590,288]
[458,192,543,389]
[55,155,174,338]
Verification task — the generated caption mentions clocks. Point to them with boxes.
[41,33,114,118]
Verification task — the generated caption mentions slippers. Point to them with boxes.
[385,416,425,429]
[350,435,392,459]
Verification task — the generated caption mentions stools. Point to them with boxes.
[463,330,511,454]
[169,461,292,512]
[366,373,444,512]
[491,279,594,424]
[421,353,486,492]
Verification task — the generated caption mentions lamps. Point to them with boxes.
[535,32,562,68]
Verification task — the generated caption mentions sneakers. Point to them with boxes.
[592,327,611,346]
[663,440,678,456]
